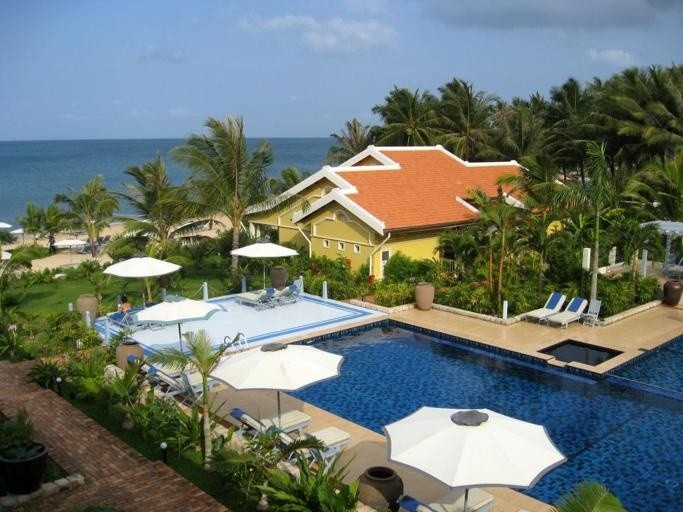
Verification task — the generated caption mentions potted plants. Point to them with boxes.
[0,404,48,495]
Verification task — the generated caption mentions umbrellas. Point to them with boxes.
[206,341,344,431]
[102,251,183,303]
[136,293,229,375]
[0,221,24,260]
[379,405,570,511]
[53,237,87,264]
[228,238,301,290]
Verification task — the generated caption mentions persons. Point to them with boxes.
[45,234,58,254]
[117,294,131,314]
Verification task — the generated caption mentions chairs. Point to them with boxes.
[277,426,350,474]
[523,290,566,325]
[582,299,601,326]
[108,306,147,332]
[396,486,496,512]
[229,406,312,442]
[140,301,165,331]
[235,284,298,310]
[155,369,223,399]
[545,298,588,328]
[78,234,112,256]
[128,355,196,384]
